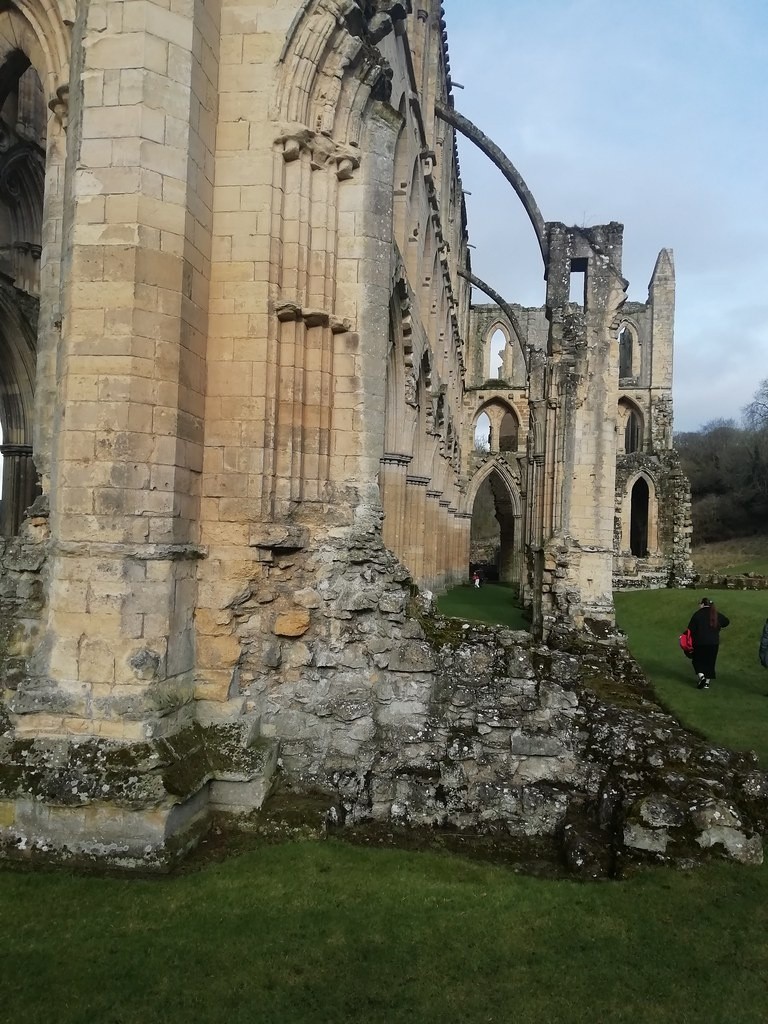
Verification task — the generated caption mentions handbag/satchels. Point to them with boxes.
[680,630,693,651]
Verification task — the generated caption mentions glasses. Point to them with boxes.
[699,603,703,605]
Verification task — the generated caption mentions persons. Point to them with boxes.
[472,572,480,588]
[683,597,730,689]
[760,618,768,669]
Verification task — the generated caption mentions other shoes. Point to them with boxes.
[704,683,710,688]
[697,676,706,689]
